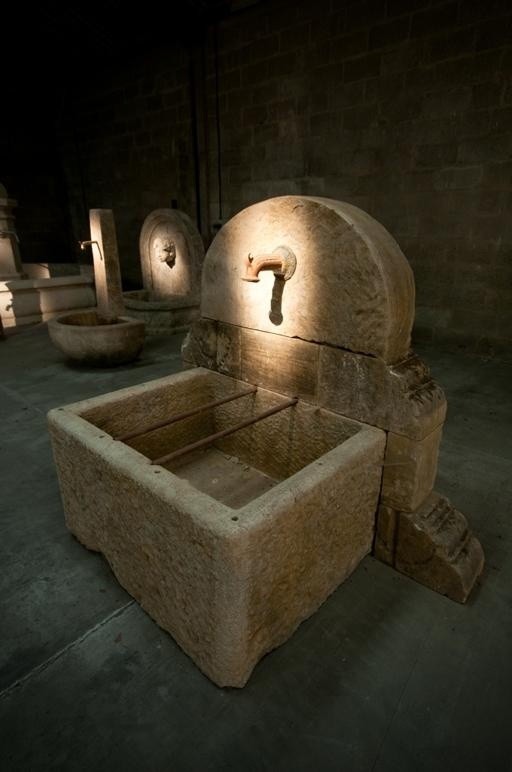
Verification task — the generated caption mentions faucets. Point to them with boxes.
[78,240,97,250]
[240,245,295,283]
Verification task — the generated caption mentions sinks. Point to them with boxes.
[46,308,146,367]
[122,290,199,335]
[47,368,385,689]
